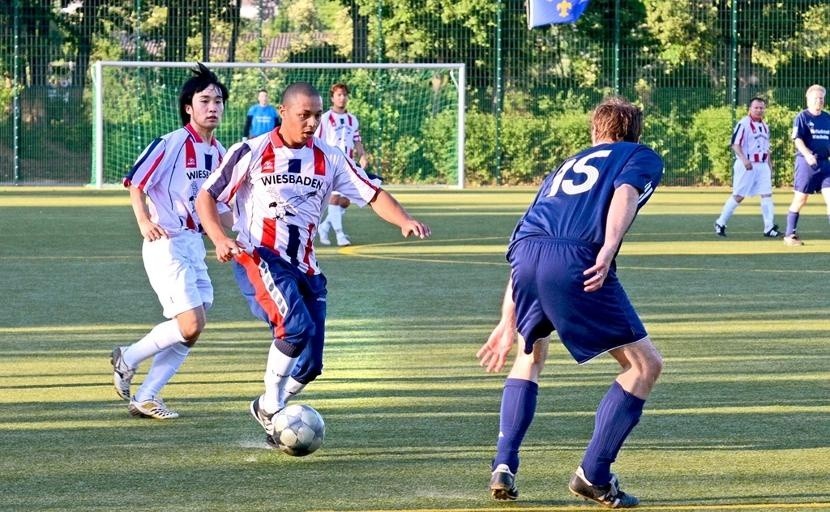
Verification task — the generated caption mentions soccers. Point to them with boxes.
[273,404,325,456]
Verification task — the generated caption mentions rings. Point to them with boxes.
[597,273,603,277]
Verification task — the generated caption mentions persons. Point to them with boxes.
[784,84,830,245]
[313,83,368,246]
[475,94,665,507]
[108,59,228,420]
[243,90,280,144]
[712,98,783,237]
[195,83,431,437]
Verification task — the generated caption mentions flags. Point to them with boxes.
[527,0,588,34]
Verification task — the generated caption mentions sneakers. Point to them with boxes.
[783,233,804,246]
[336,233,352,246]
[108,346,139,401]
[128,394,179,420]
[712,220,728,238]
[763,224,786,239]
[568,464,640,508]
[249,393,286,449]
[317,225,331,245]
[488,464,519,500]
